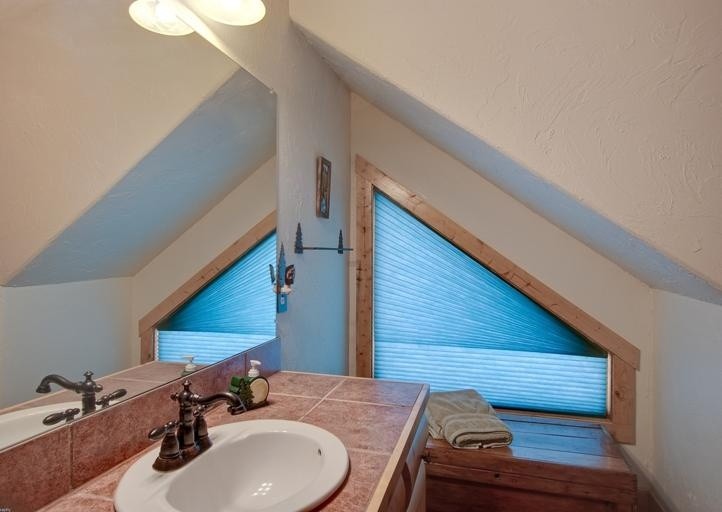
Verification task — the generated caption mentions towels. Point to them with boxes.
[424,388,512,450]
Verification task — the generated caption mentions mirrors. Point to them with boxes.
[1,3,280,452]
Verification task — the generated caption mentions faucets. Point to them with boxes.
[36,369,102,416]
[169,380,246,459]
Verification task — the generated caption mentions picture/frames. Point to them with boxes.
[314,156,332,218]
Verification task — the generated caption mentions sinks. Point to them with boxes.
[113,419,349,512]
[0,400,121,448]
